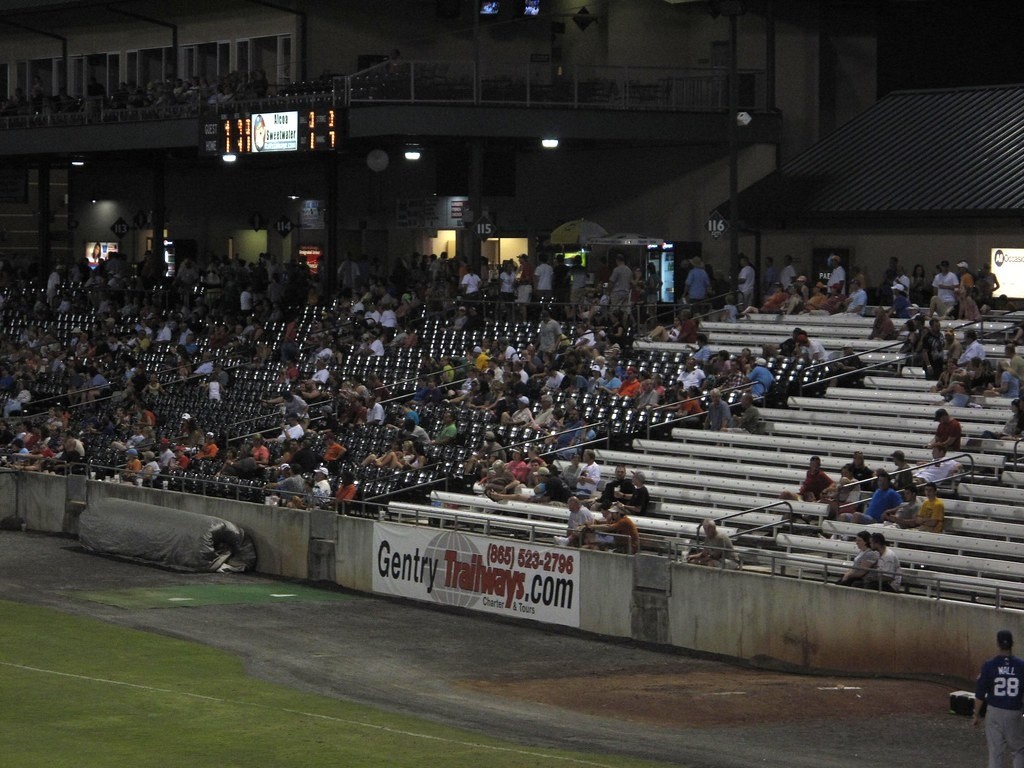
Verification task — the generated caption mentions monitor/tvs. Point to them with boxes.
[84,242,120,270]
[479,0,540,17]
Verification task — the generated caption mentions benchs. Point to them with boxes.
[385,306,1024,610]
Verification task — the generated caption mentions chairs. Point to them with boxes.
[2,265,839,514]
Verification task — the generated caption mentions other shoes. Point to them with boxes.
[554,536,567,547]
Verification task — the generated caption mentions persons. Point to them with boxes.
[687,519,741,569]
[0,247,867,511]
[0,69,268,126]
[889,317,1024,442]
[86,242,102,263]
[773,444,965,533]
[554,465,649,553]
[366,49,409,83]
[837,530,902,593]
[971,630,1024,768]
[924,409,962,451]
[869,254,1017,341]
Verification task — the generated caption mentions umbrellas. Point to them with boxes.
[551,218,608,255]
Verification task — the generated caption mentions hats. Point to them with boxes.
[0,239,1024,524]
[996,630,1013,644]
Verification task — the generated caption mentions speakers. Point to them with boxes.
[738,112,753,126]
